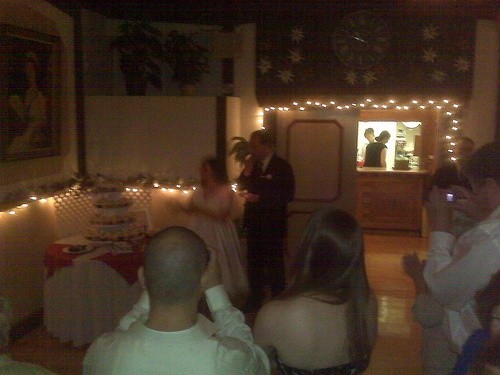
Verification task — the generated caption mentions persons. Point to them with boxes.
[177,157,252,321]
[253,205,379,375]
[362,128,500,375]
[81,227,274,375]
[234,130,295,310]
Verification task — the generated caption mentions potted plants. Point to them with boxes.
[110,21,162,96]
[161,30,209,96]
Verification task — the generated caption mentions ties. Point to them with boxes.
[258,160,264,177]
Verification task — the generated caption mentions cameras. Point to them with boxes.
[445,193,457,204]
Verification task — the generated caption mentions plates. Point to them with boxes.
[62,243,93,253]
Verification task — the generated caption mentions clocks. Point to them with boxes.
[332,10,392,70]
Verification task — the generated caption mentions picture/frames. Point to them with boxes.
[0,24,62,163]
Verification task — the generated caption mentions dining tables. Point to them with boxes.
[43,232,155,347]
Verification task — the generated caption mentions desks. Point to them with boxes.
[357,167,429,231]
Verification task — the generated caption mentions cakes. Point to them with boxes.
[84,178,148,239]
